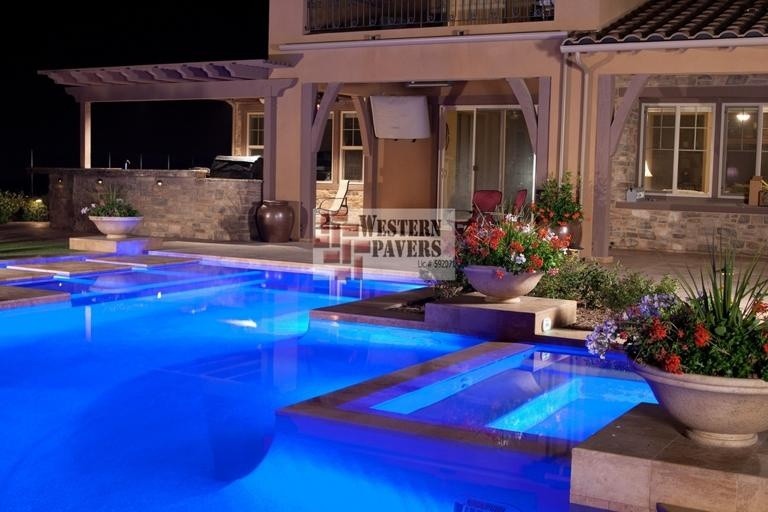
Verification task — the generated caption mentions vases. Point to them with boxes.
[255,199,295,243]
[460,264,544,304]
[89,216,144,239]
[625,362,768,448]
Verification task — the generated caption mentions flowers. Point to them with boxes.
[81,184,144,217]
[452,171,585,281]
[584,223,768,387]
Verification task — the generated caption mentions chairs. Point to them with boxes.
[316,179,350,229]
